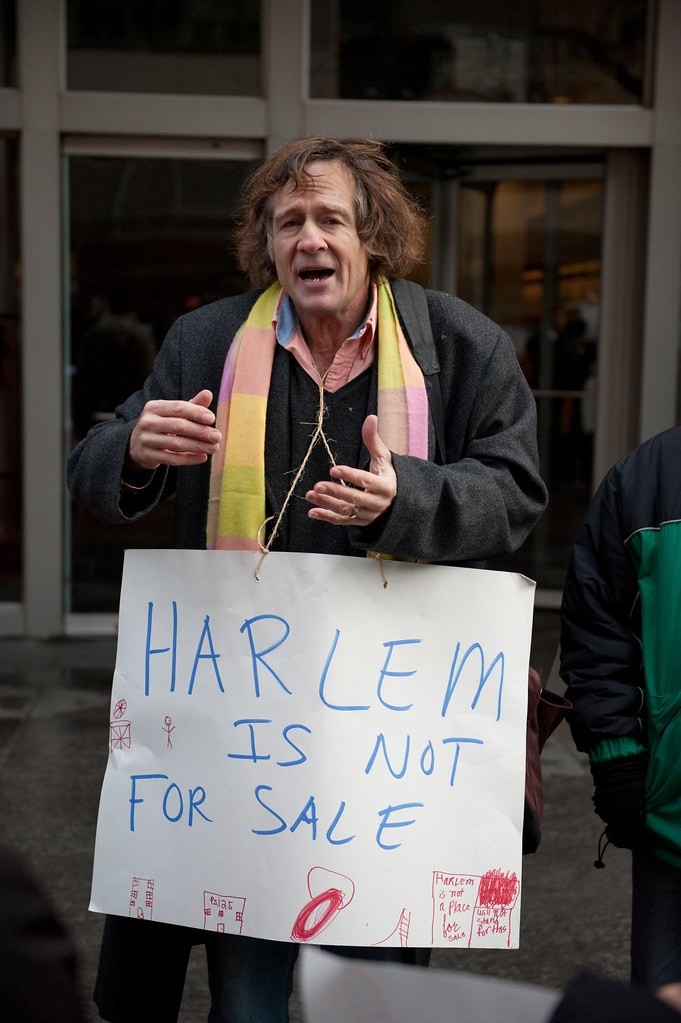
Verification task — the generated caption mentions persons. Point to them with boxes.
[562,426,681,1023]
[523,315,601,580]
[65,138,544,1023]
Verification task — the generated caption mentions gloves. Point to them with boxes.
[589,747,646,853]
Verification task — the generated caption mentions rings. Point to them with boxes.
[349,505,358,519]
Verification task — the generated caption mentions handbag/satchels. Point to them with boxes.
[523,667,574,854]
